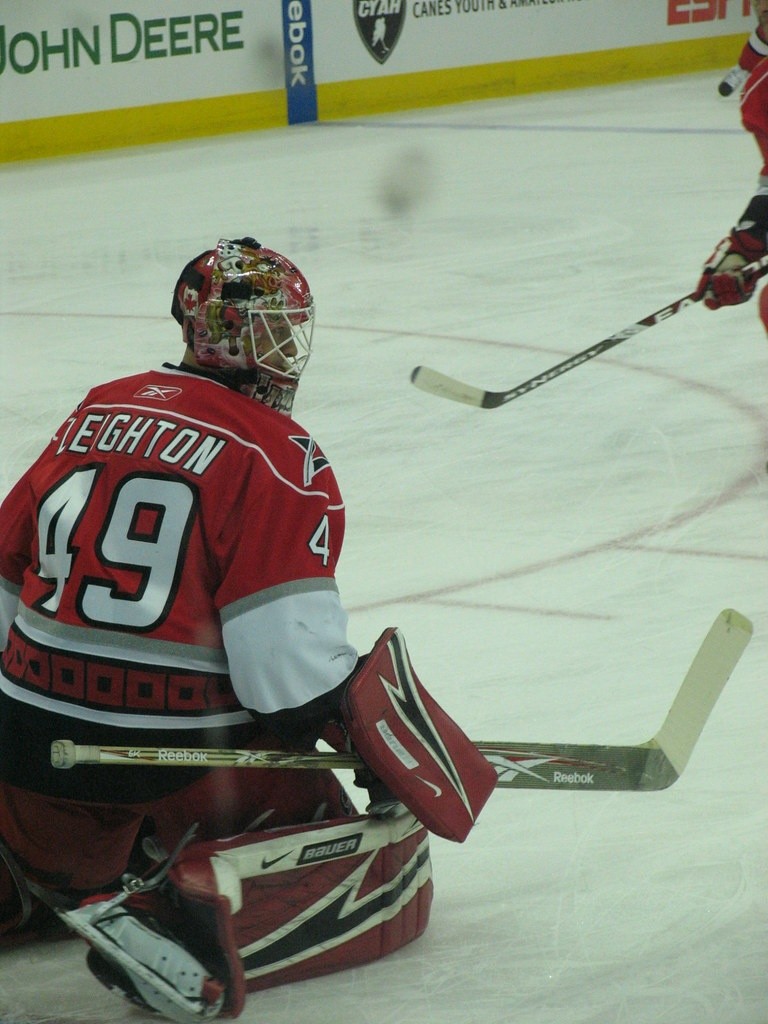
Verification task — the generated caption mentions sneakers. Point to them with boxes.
[56,892,224,1023]
[719,63,749,96]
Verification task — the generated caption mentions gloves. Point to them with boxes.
[698,219,768,311]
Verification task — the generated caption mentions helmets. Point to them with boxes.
[171,237,316,384]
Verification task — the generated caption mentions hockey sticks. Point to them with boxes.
[48,606,756,796]
[406,253,768,408]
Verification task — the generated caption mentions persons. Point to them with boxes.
[0,236,497,1023]
[698,0,768,333]
